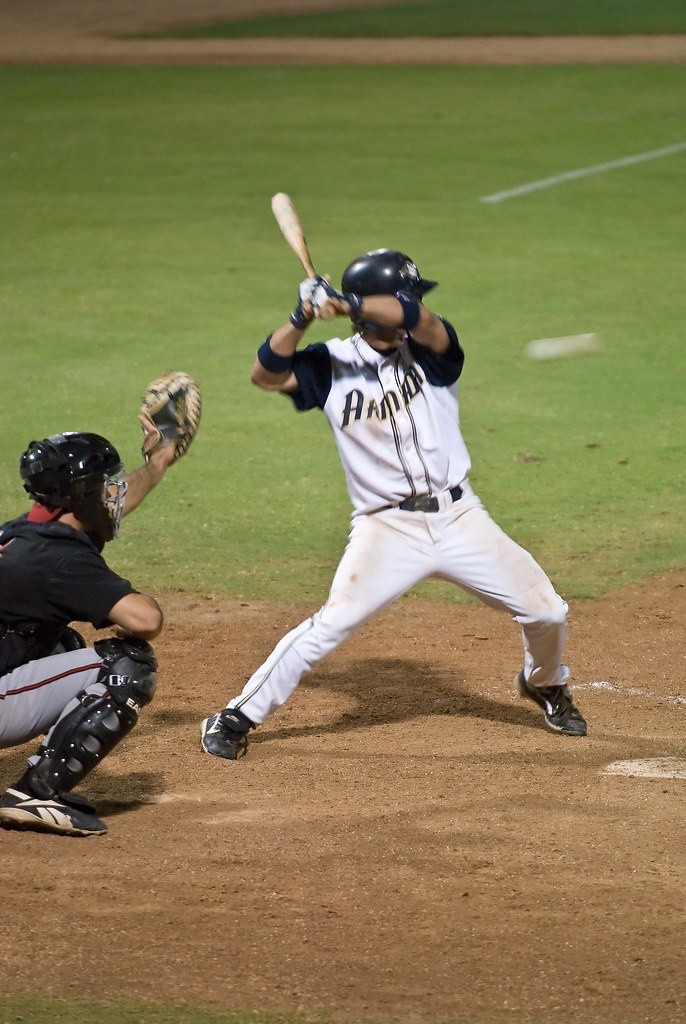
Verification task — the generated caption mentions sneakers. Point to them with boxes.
[201,708,255,760]
[1,783,109,835]
[514,669,587,735]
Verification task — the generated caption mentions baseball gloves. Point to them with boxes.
[139,370,202,469]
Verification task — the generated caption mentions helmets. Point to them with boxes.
[341,247,439,298]
[20,430,123,510]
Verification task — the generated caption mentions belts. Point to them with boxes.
[383,487,462,513]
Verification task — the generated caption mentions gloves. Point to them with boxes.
[289,273,331,329]
[310,279,360,321]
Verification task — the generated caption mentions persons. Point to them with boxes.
[200,249,586,760]
[0,370,201,837]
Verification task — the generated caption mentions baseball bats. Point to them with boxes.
[270,192,335,322]
